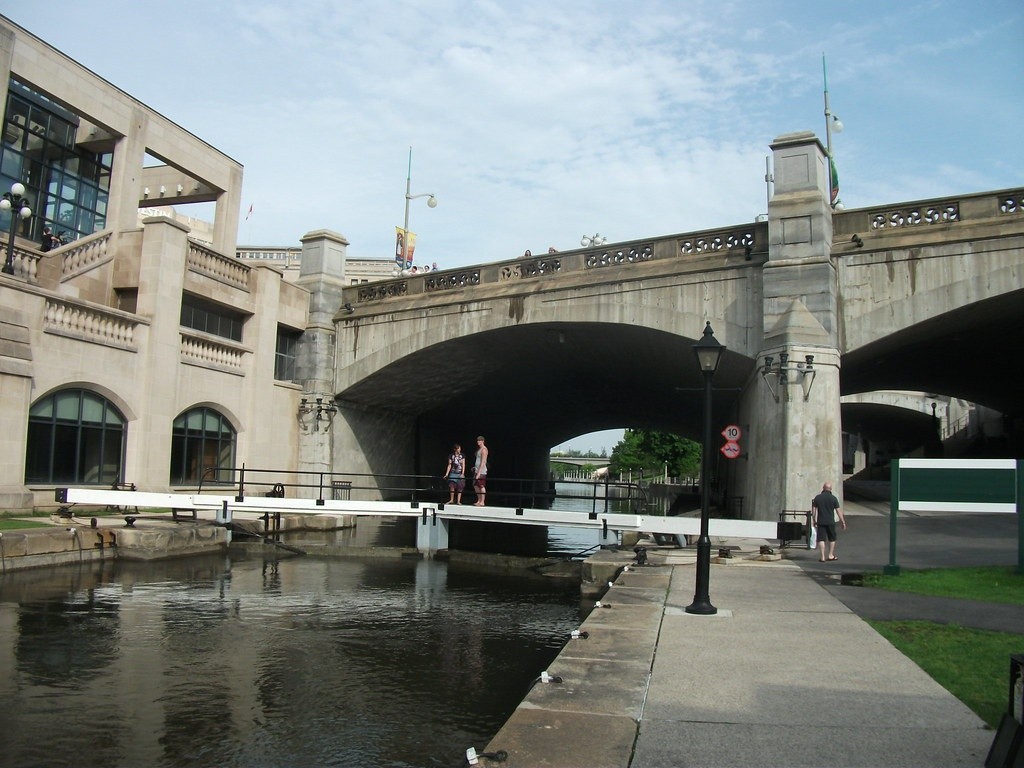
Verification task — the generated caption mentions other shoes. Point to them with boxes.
[474,502,484,507]
[447,501,462,505]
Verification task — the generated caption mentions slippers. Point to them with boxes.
[819,558,825,562]
[828,556,838,560]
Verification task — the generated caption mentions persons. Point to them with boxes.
[40,227,69,252]
[471,436,488,507]
[443,444,466,505]
[546,247,559,273]
[518,249,536,276]
[407,262,440,284]
[812,482,846,563]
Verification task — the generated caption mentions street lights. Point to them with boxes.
[688,320,722,614]
[399,194,436,275]
[826,115,846,213]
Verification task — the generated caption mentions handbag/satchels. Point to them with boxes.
[810,523,817,549]
